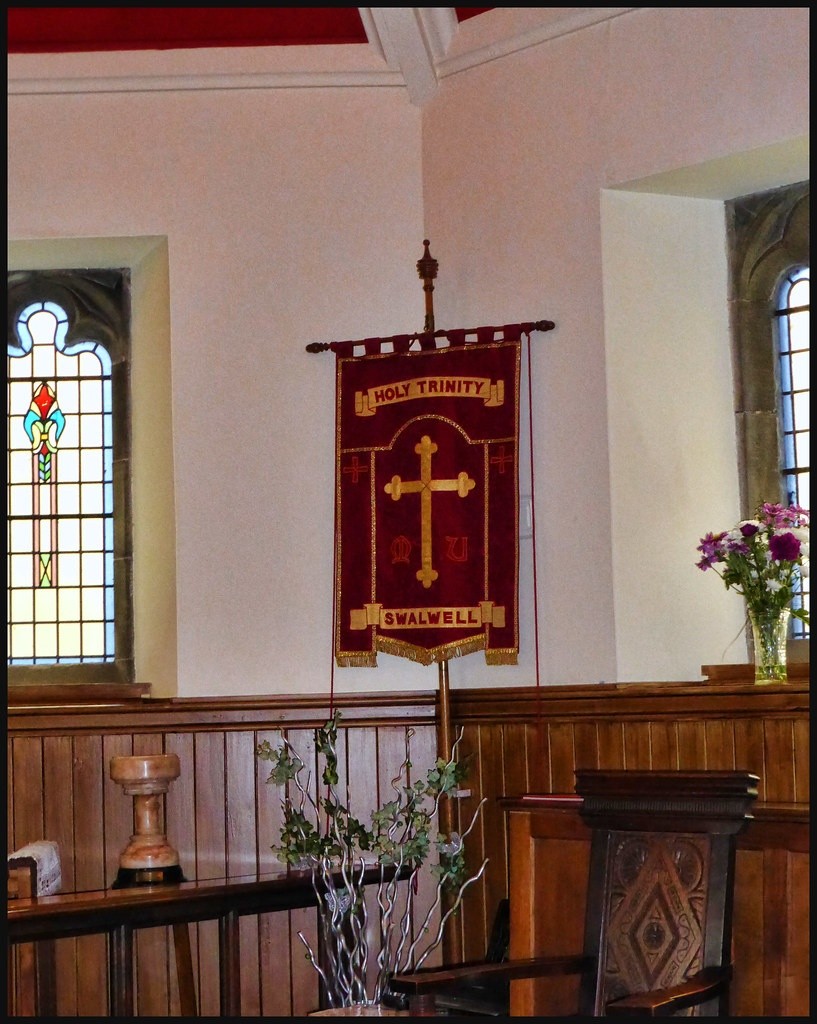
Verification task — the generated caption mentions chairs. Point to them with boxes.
[386,767,761,1018]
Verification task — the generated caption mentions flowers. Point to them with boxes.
[696,500,811,661]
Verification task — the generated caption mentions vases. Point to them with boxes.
[748,608,791,683]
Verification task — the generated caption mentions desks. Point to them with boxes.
[5,864,413,1017]
